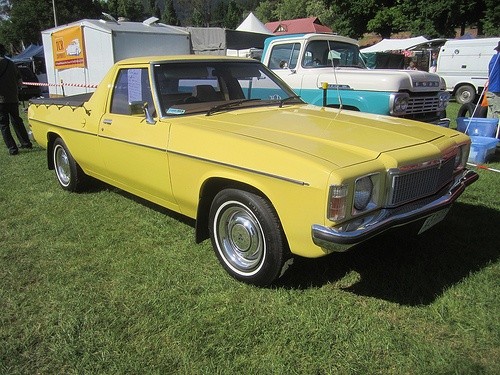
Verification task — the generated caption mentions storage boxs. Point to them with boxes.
[455,117,499,138]
[467,134,500,165]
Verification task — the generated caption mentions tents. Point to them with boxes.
[359,36,431,73]
[227,12,271,59]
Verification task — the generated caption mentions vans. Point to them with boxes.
[435,37,500,104]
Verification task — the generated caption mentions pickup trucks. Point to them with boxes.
[27,53,480,287]
[177,32,449,130]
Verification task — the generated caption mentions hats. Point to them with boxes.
[493,41,500,51]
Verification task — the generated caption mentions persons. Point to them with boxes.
[429,51,438,73]
[486,42,500,118]
[0,43,32,155]
[406,61,418,69]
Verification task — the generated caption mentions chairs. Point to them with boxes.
[190,84,221,103]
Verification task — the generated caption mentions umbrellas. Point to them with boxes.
[406,36,455,73]
